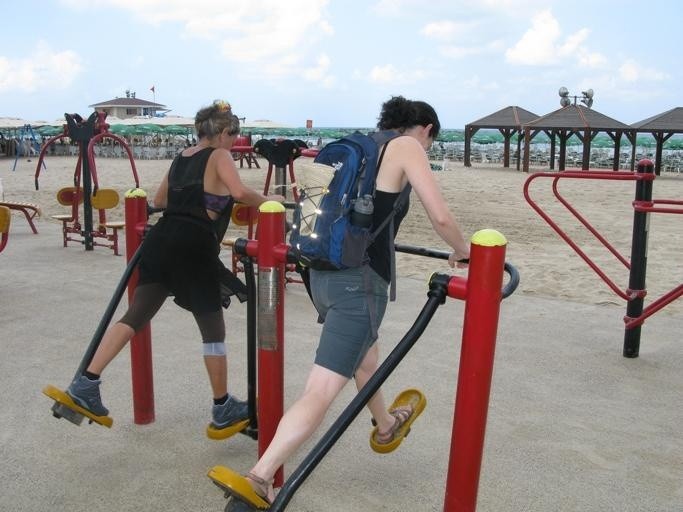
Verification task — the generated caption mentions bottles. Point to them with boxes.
[350,194,374,227]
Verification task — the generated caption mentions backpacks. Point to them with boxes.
[285,130,412,271]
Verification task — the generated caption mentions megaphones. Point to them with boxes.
[582,88,594,99]
[560,98,570,107]
[557,87,569,96]
[581,98,594,107]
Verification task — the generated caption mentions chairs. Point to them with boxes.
[17,138,196,160]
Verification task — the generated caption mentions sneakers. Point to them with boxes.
[211,393,249,429]
[67,372,109,416]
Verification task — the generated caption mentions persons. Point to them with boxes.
[69,105,284,429]
[242,94,471,504]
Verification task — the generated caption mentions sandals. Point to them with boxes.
[375,404,414,444]
[244,473,275,504]
